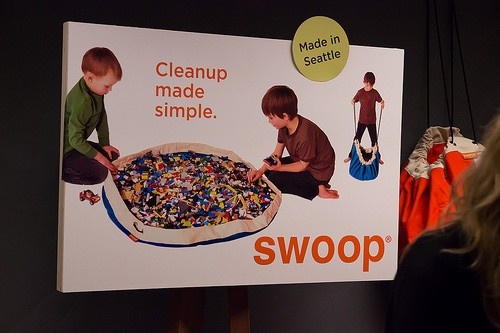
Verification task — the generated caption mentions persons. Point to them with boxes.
[63,47,123,185]
[344,72,384,164]
[388,114,499,332]
[247,85,339,200]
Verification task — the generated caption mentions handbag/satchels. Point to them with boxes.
[398,0,488,268]
